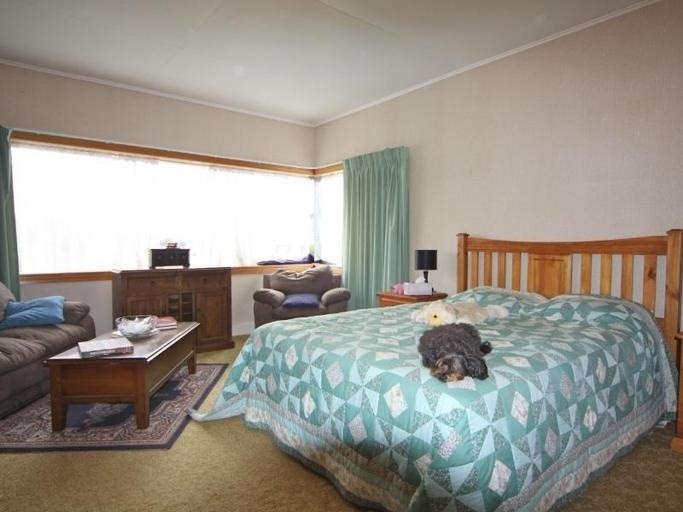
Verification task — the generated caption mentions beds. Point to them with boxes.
[185,226,683,511]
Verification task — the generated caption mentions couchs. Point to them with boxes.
[0,281,96,422]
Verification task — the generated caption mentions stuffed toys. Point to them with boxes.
[409,297,510,325]
[417,322,492,382]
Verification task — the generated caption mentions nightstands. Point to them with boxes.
[669,329,682,457]
[376,290,448,312]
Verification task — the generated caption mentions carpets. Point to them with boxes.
[0,362,229,452]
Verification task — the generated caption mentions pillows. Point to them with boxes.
[276,264,334,296]
[1,294,66,330]
[281,295,319,308]
[270,276,316,295]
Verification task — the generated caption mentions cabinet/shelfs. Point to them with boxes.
[109,266,235,354]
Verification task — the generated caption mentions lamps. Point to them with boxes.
[412,248,437,292]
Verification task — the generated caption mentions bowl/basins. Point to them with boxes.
[114,315,158,339]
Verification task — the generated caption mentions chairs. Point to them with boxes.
[251,274,350,328]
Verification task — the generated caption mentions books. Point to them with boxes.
[155,315,179,331]
[77,337,135,358]
